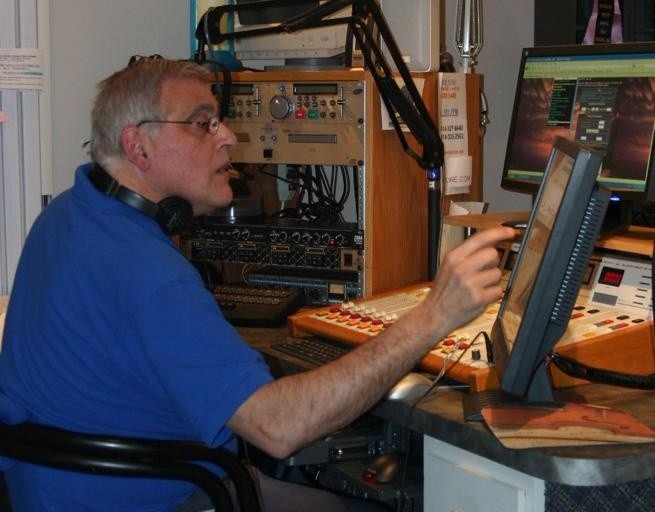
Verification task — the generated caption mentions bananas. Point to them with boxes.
[268,332,352,371]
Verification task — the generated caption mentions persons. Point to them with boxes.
[0,60,517,512]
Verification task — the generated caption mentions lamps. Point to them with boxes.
[0,420,261,512]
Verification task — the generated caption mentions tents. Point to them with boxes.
[81,161,195,243]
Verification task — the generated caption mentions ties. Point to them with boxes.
[499,42,655,241]
[463,133,614,430]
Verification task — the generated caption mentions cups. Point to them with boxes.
[133,115,222,136]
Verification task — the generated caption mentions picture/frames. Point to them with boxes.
[219,320,655,507]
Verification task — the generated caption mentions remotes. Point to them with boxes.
[389,373,437,406]
[366,448,408,487]
